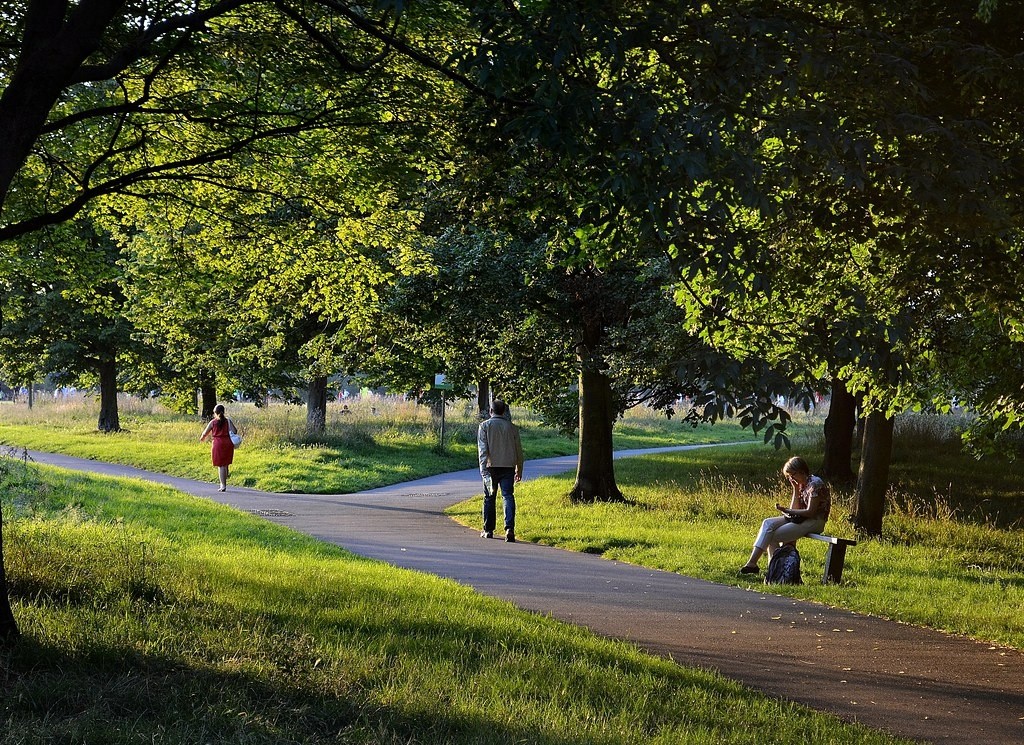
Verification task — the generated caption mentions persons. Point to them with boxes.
[340,405,352,416]
[54,384,77,400]
[338,386,373,404]
[371,408,379,417]
[773,390,824,414]
[477,401,523,542]
[200,405,237,491]
[740,457,830,574]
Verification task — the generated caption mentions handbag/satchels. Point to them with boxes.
[784,512,809,524]
[228,418,241,448]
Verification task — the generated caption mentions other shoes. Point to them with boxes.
[480,531,493,538]
[741,566,760,573]
[218,487,226,491]
[505,528,516,542]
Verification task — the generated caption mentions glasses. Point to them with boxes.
[788,471,796,478]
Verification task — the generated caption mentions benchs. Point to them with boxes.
[800,533,857,584]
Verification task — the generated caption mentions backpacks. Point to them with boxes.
[765,543,804,585]
